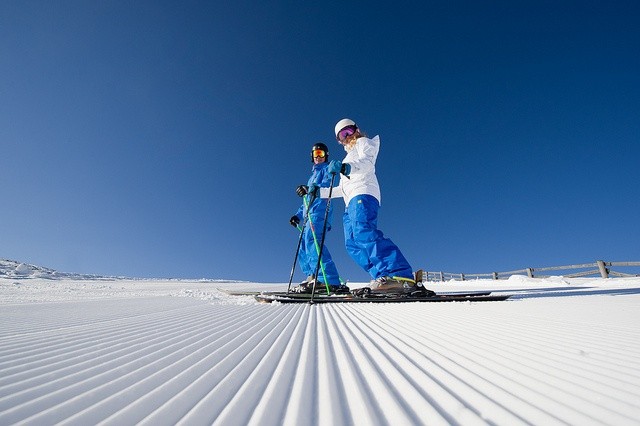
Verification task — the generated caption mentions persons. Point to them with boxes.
[308,118,416,296]
[289,143,346,294]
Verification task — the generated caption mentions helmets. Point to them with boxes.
[311,143,329,163]
[334,118,359,139]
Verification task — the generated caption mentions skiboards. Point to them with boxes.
[255,291,512,302]
[229,292,354,298]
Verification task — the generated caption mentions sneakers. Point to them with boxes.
[295,280,311,294]
[373,278,415,292]
[307,278,341,292]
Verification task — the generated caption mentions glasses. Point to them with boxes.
[337,125,356,140]
[313,149,326,158]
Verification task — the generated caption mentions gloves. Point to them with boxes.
[328,159,350,175]
[308,183,320,197]
[290,215,300,227]
[295,184,309,197]
